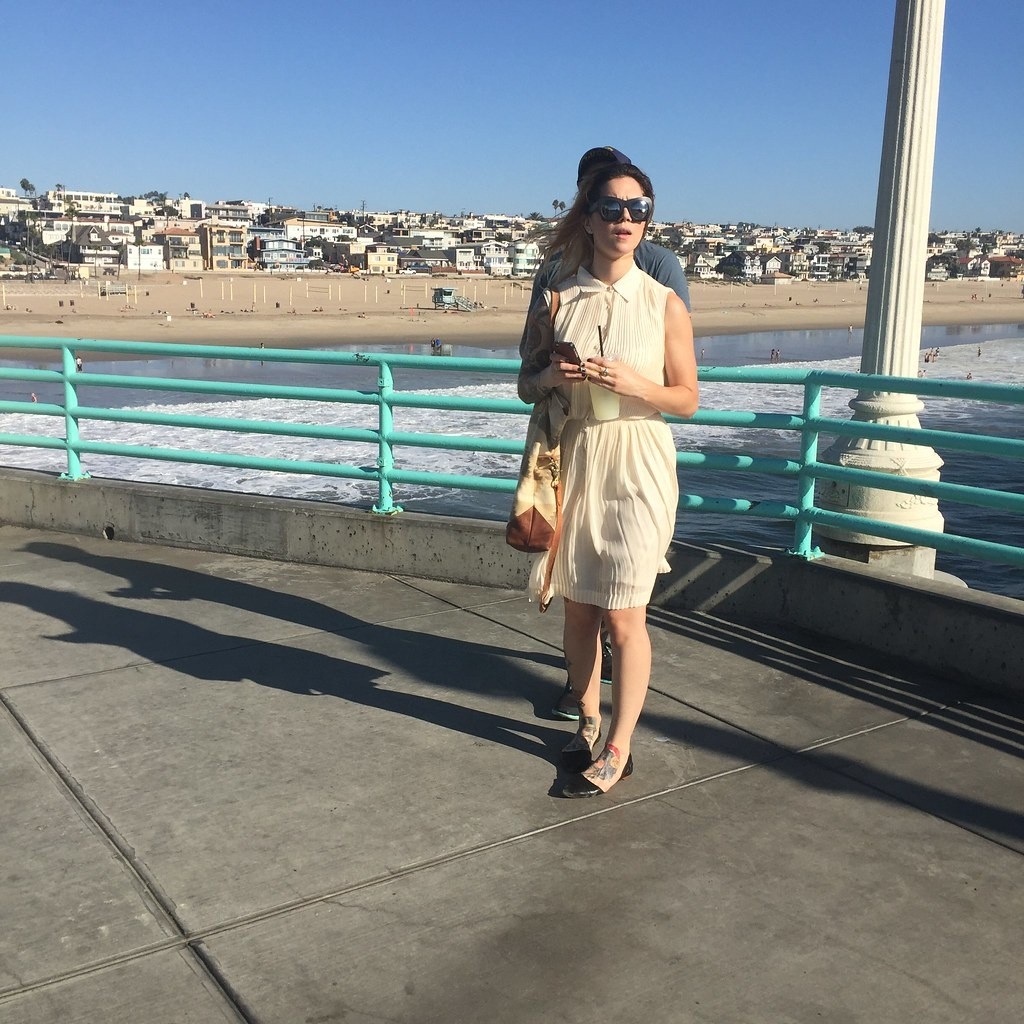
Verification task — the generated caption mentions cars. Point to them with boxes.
[9,266,23,271]
[44,273,57,279]
[25,273,40,280]
[3,274,26,280]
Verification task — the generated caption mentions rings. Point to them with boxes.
[602,366,608,378]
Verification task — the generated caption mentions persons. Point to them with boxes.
[517,164,702,801]
[519,144,692,722]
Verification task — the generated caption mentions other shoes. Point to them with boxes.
[554,751,633,798]
[561,728,601,773]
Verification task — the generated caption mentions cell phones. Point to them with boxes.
[554,340,582,367]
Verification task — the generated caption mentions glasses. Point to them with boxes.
[588,196,652,222]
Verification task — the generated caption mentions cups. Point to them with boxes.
[587,355,621,422]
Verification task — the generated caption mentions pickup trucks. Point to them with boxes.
[399,268,416,275]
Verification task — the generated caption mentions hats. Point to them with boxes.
[578,145,630,179]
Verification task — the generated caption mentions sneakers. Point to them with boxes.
[551,678,579,719]
[600,632,614,684]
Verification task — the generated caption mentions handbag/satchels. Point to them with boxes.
[505,388,573,552]
